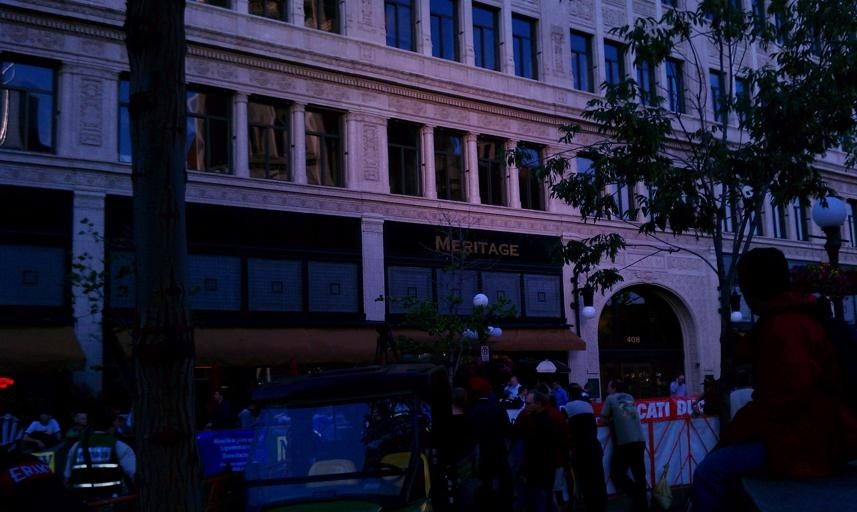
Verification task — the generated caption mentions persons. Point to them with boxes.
[670,375,686,397]
[432,387,491,511]
[691,248,857,512]
[598,379,647,512]
[576,382,591,400]
[62,409,138,502]
[206,387,235,431]
[378,345,395,360]
[552,381,566,408]
[0,452,73,512]
[241,403,257,428]
[503,377,522,398]
[516,390,558,512]
[466,363,514,511]
[516,385,527,405]
[560,383,606,512]
[21,413,60,449]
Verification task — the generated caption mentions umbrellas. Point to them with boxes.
[527,358,571,374]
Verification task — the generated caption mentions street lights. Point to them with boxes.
[464,292,503,358]
[812,196,847,317]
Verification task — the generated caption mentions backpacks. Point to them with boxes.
[784,295,857,414]
